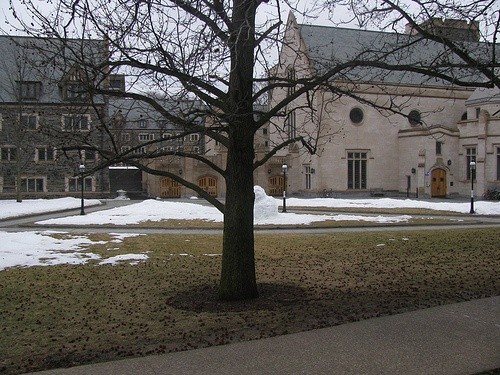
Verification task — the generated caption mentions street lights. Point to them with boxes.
[80,162,86,215]
[469,160,476,214]
[281,163,288,213]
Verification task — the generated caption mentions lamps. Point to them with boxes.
[412,168,415,173]
[448,160,451,165]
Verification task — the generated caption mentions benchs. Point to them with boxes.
[371,188,386,197]
